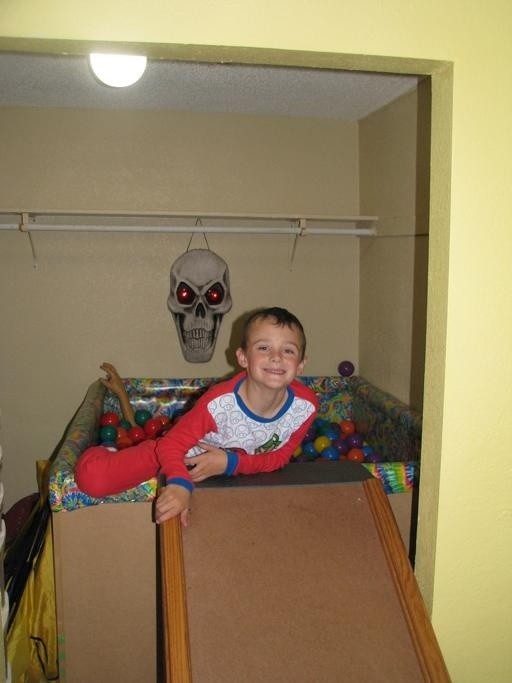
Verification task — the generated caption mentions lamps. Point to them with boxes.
[89,51,149,87]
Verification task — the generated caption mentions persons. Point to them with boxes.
[99,363,137,428]
[75,308,319,525]
